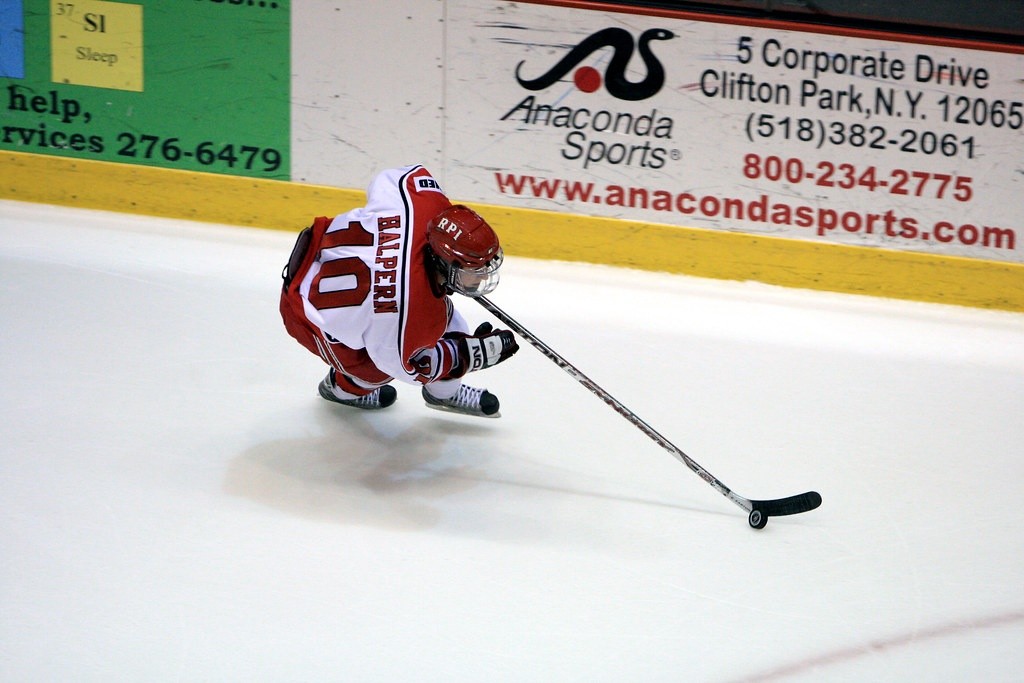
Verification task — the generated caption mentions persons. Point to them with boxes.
[279,164,519,419]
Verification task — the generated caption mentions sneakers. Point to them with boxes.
[318,365,396,409]
[422,382,501,419]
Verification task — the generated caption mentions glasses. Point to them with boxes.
[466,247,504,276]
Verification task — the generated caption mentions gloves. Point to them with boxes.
[448,321,519,378]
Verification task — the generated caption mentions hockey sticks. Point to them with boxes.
[473,294,822,516]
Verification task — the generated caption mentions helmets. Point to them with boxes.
[427,204,504,297]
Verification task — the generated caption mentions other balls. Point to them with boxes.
[748,509,767,530]
